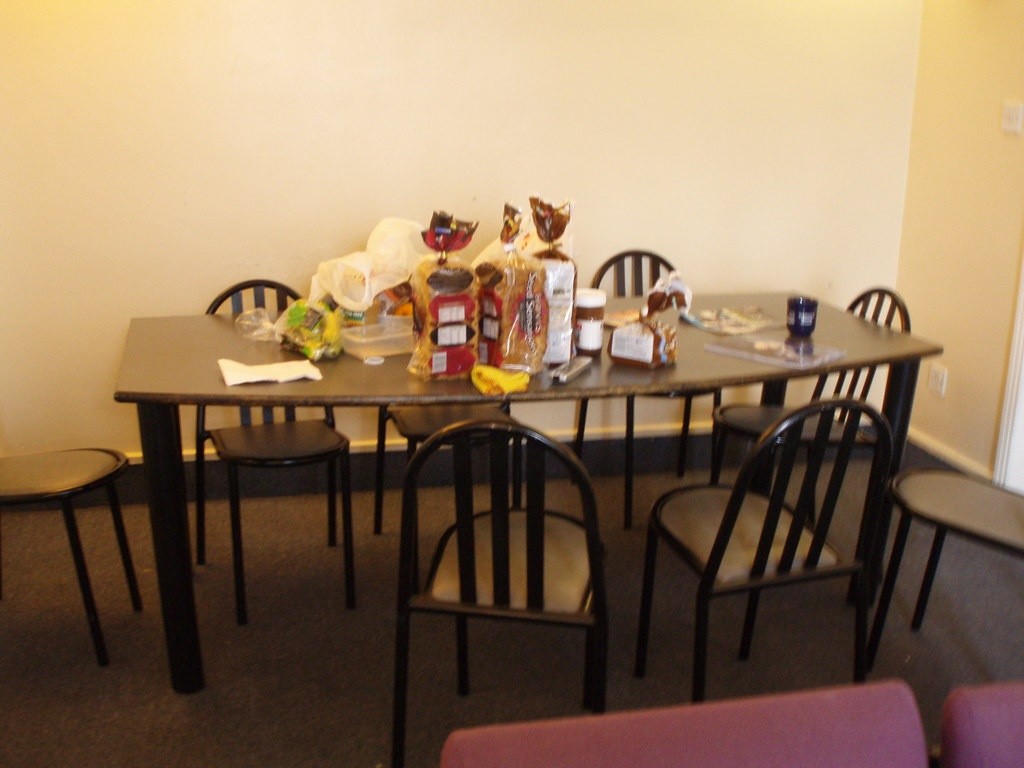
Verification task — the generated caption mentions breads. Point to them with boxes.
[609,320,678,369]
[408,250,576,380]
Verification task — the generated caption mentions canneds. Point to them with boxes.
[573,288,607,356]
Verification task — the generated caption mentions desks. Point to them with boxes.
[112,291,941,689]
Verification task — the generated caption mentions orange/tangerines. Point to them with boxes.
[381,290,413,318]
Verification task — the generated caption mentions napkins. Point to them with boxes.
[218,359,323,387]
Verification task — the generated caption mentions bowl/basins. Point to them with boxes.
[342,321,414,358]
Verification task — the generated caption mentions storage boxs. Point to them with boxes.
[340,325,415,359]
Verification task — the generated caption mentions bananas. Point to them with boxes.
[281,297,342,361]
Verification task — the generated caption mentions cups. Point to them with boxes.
[786,299,819,338]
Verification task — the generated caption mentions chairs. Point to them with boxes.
[635,402,892,706]
[374,401,521,534]
[712,285,911,528]
[0,448,142,667]
[572,249,722,529]
[196,280,356,625]
[392,420,609,768]
[867,467,1024,673]
[441,678,928,768]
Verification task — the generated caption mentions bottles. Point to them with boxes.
[573,288,606,359]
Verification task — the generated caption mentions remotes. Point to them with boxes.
[548,355,593,383]
